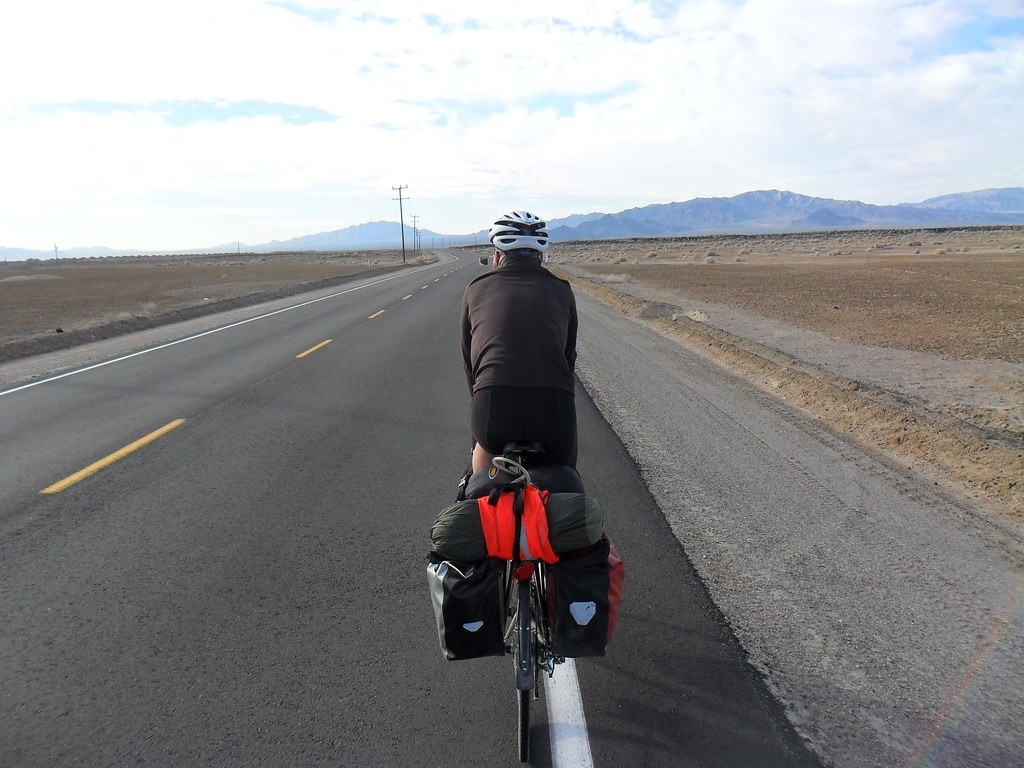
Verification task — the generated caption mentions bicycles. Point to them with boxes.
[502,439,556,764]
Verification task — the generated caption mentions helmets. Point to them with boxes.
[489,210,549,252]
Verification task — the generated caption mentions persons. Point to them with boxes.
[459,210,578,471]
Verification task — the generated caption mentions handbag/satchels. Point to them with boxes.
[427,492,623,661]
